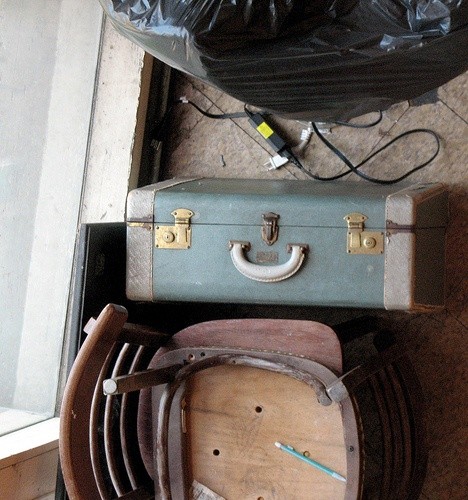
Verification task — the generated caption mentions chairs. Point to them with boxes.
[59,302,394,500]
[102,321,430,498]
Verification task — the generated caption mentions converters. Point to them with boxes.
[246,113,287,156]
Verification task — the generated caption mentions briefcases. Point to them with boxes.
[125,178,448,315]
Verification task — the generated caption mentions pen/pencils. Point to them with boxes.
[274,441,347,482]
